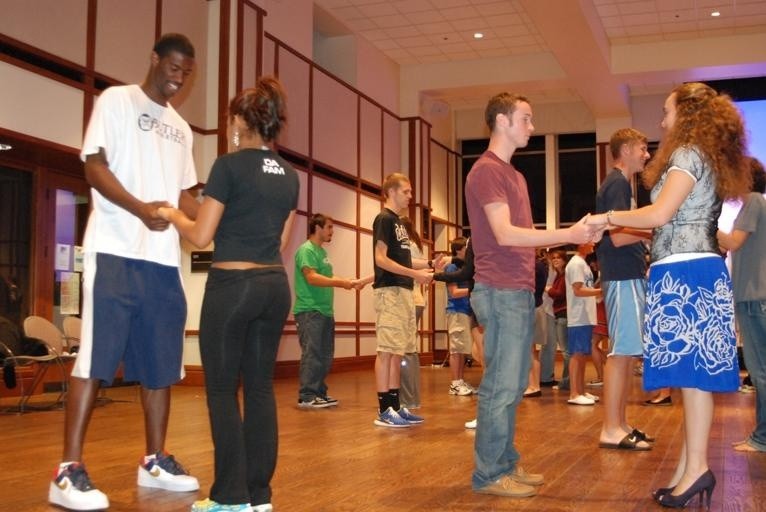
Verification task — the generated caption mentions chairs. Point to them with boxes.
[18,315,77,411]
[0,317,66,413]
[55,317,112,408]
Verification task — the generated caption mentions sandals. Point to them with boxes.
[731,440,748,445]
[734,442,761,451]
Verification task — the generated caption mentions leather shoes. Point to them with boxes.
[588,380,604,386]
[541,381,557,387]
[642,396,672,405]
[522,391,543,397]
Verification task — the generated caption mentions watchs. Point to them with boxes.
[606,209,615,227]
[427,261,433,268]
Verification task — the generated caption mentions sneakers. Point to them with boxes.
[463,383,478,394]
[374,407,412,427]
[137,455,200,493]
[508,464,545,486]
[397,404,425,423]
[475,475,536,498]
[190,496,253,512]
[449,382,471,396]
[48,459,110,511]
[465,418,477,429]
[298,394,331,407]
[739,385,757,393]
[252,502,273,512]
[324,397,338,406]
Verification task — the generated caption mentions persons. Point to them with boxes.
[523,246,548,398]
[539,257,558,386]
[594,127,658,456]
[584,254,609,386]
[562,241,601,406]
[439,237,478,432]
[157,73,300,511]
[714,156,765,455]
[735,372,755,393]
[442,237,479,396]
[463,90,602,496]
[43,35,200,511]
[352,217,426,411]
[584,83,754,511]
[292,211,356,409]
[544,249,571,392]
[370,172,444,430]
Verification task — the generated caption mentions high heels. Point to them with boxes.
[653,485,679,501]
[656,470,716,509]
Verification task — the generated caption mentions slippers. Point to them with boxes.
[598,434,653,450]
[631,429,655,442]
[568,395,595,405]
[584,392,599,401]
